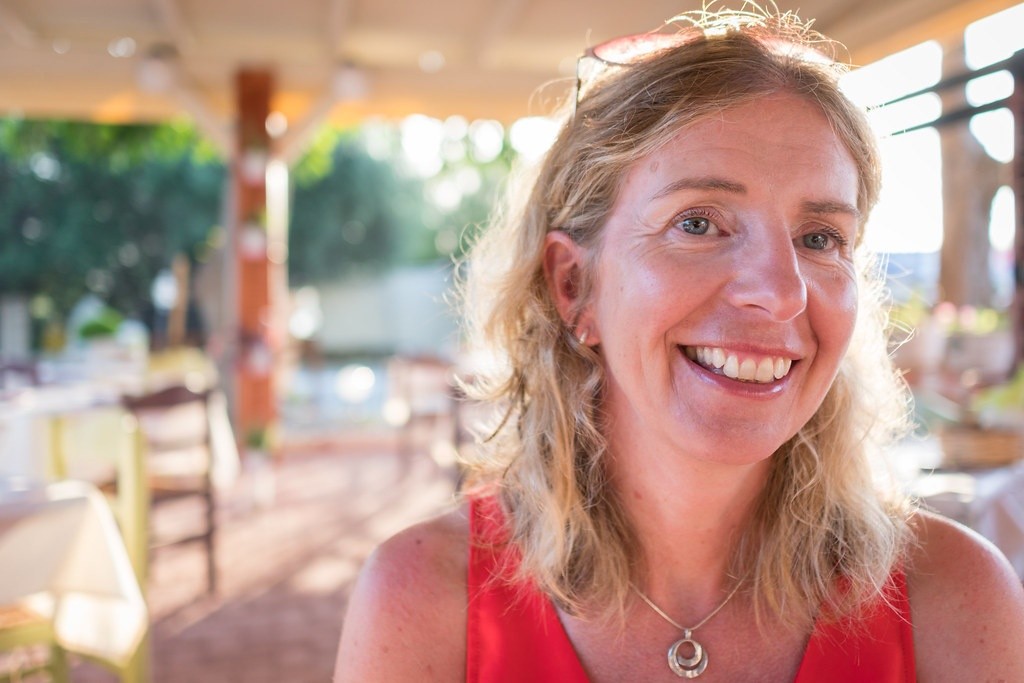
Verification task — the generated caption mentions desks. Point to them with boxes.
[0,345,240,515]
[902,436,1024,584]
[0,471,153,683]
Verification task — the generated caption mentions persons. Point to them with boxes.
[333,0,1024,683]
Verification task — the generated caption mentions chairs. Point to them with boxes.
[94,385,220,592]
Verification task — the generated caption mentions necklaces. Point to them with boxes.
[626,562,754,678]
